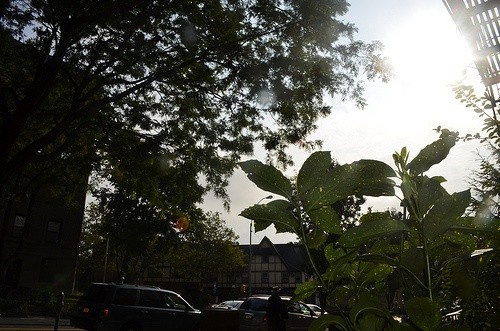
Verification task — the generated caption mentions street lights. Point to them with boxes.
[248,195,273,296]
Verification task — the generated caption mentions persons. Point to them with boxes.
[265,286,291,331]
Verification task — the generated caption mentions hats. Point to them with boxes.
[271,286,281,292]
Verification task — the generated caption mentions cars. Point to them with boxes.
[306,304,328,317]
[214,300,245,311]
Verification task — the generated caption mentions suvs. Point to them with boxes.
[70,282,201,331]
[238,296,331,331]
